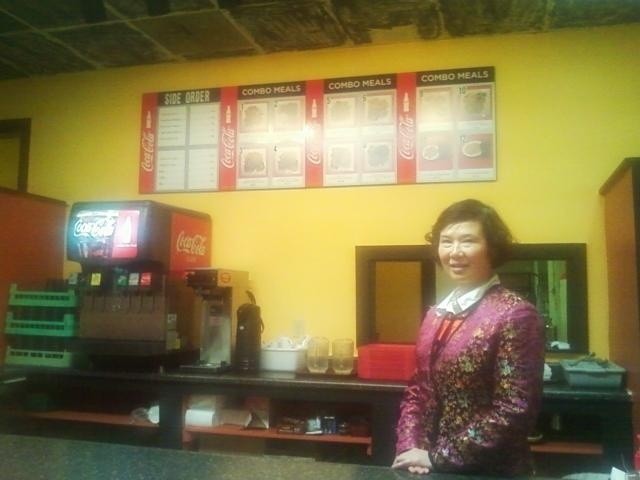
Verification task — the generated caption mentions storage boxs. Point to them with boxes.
[358,343,417,381]
[259,341,306,372]
[560,359,626,389]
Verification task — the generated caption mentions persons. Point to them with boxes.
[391,199,546,476]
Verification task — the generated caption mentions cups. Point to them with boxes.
[306,339,328,375]
[331,338,354,374]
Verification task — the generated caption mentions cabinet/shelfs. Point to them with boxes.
[177,378,397,466]
[533,392,632,473]
[0,365,176,449]
